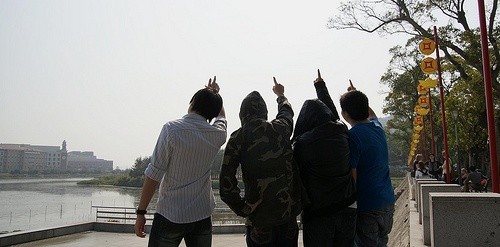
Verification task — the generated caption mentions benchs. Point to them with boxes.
[471,178,490,192]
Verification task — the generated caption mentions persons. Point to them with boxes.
[339,79,395,247]
[134,75,227,247]
[399,151,489,193]
[291,69,358,247]
[219,77,299,247]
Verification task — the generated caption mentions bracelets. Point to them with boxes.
[135,209,147,215]
